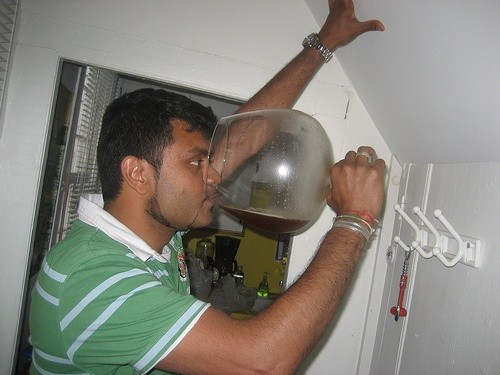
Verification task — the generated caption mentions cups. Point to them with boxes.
[205,108,335,234]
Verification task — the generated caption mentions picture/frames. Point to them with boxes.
[196,204,246,237]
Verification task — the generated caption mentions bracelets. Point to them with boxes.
[332,209,379,242]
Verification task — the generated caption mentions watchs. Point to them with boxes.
[302,33,333,62]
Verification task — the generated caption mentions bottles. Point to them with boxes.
[233,265,244,286]
[256,272,269,297]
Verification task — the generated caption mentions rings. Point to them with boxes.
[356,152,372,163]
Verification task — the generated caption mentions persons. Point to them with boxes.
[29,0,387,375]
